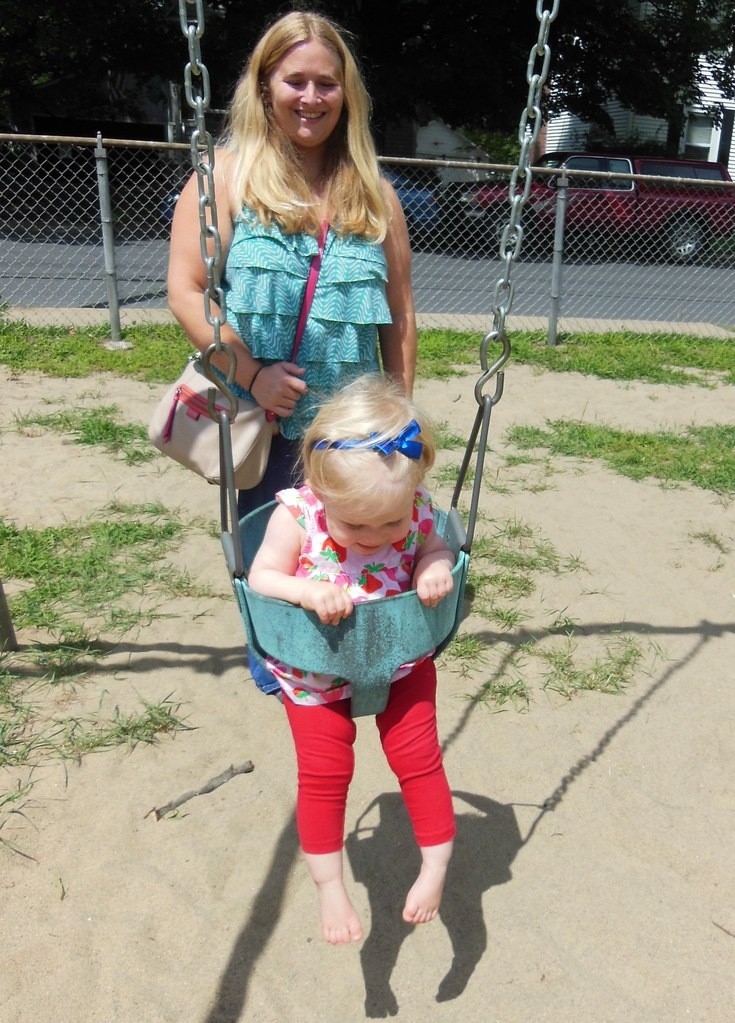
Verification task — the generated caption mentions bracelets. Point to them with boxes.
[248,365,264,395]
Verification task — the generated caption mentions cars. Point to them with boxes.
[165,163,440,255]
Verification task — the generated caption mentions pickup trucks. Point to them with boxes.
[445,149,734,269]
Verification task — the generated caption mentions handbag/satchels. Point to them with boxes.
[147,358,275,492]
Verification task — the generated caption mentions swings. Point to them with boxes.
[173,1,567,719]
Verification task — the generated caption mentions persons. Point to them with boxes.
[148,11,415,523]
[247,374,458,944]
